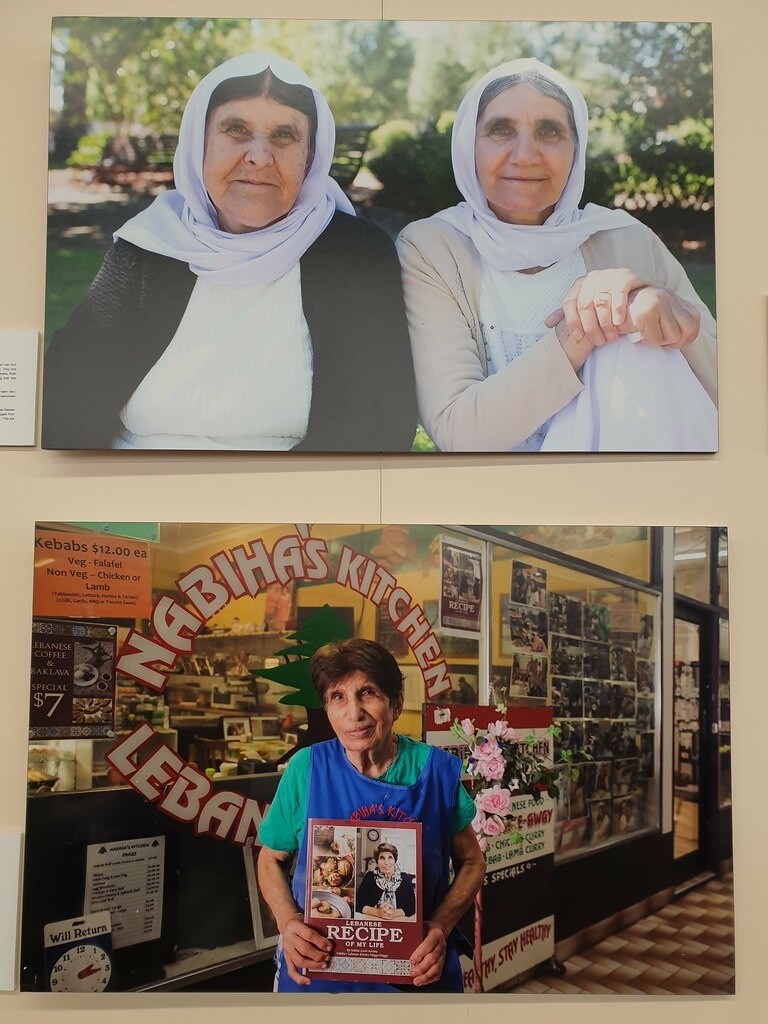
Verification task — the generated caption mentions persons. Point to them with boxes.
[459,561,481,601]
[258,638,487,993]
[43,52,417,451]
[356,843,416,920]
[395,57,718,452]
[509,561,655,853]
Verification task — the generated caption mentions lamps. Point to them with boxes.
[674,547,727,560]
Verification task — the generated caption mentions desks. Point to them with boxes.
[168,701,281,771]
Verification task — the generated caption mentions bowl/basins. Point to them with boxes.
[312,891,351,920]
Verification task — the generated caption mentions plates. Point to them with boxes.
[74,663,98,686]
[97,681,108,691]
[102,673,112,681]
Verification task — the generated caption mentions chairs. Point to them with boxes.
[194,736,228,773]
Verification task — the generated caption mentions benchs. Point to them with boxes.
[65,120,380,199]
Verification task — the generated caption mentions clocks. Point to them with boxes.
[367,830,379,841]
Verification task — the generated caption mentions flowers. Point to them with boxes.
[447,702,597,852]
